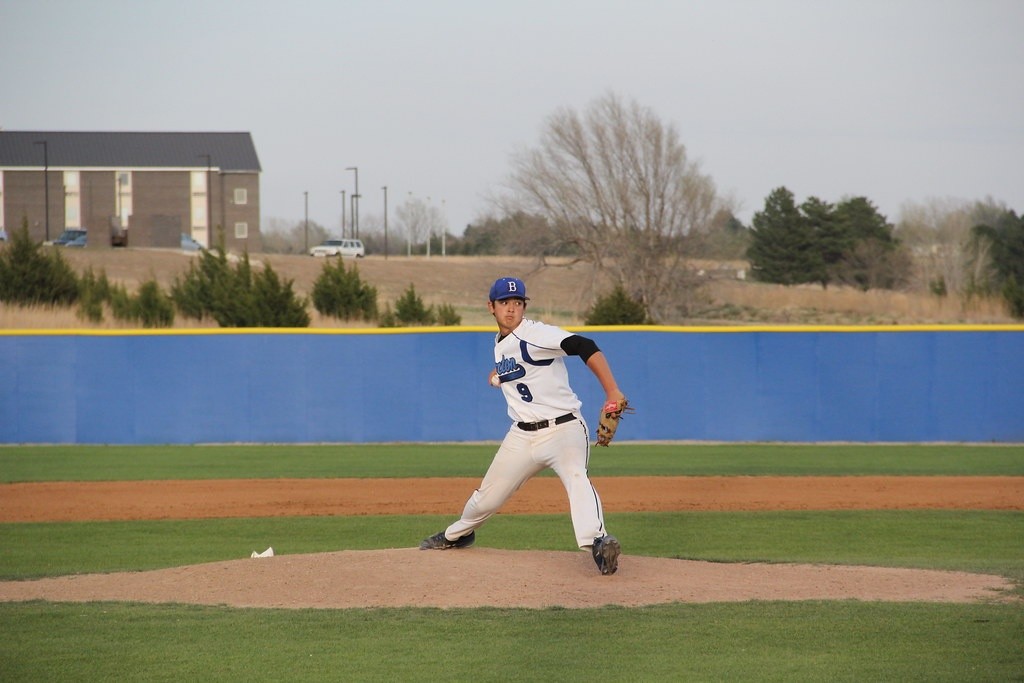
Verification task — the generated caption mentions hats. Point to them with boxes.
[488,278,530,302]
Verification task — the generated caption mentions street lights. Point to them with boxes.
[301,191,309,251]
[345,167,359,240]
[340,191,347,236]
[382,184,389,260]
[34,139,50,241]
[351,194,363,240]
[197,152,214,250]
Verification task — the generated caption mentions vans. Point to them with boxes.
[53,229,88,247]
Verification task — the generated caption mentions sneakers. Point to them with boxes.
[419,528,476,551]
[591,534,622,576]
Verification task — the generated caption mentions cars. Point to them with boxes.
[179,234,203,252]
[0,227,7,243]
[63,235,122,247]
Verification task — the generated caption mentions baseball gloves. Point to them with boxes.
[593,395,636,448]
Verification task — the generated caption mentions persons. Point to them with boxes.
[419,277,627,576]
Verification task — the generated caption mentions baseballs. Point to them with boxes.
[491,375,499,387]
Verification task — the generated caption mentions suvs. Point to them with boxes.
[310,239,366,257]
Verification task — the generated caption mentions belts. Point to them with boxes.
[517,412,577,431]
